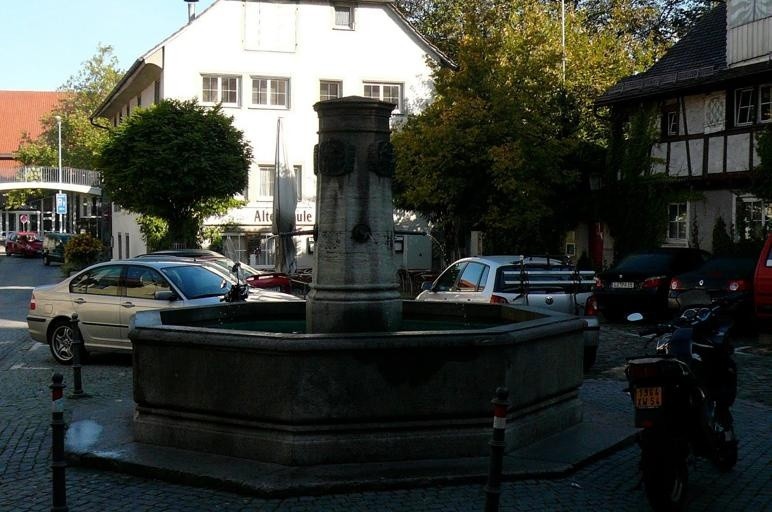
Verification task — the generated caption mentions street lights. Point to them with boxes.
[53,115,63,233]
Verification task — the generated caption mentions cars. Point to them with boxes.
[133,249,292,297]
[416,255,600,364]
[666,242,765,328]
[5,232,45,258]
[593,248,714,323]
[27,260,303,364]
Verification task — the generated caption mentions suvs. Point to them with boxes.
[42,233,76,265]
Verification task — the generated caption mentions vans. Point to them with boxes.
[755,233,772,322]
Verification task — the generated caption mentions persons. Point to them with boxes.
[576,250,592,268]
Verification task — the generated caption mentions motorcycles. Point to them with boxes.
[624,281,754,512]
